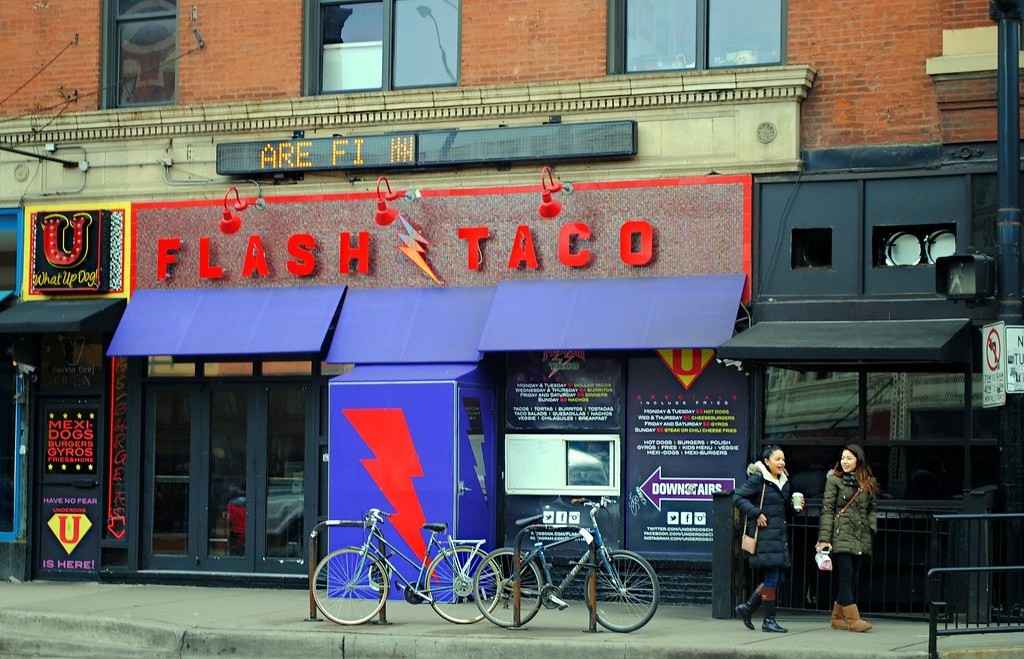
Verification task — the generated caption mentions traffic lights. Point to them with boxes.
[932,254,997,302]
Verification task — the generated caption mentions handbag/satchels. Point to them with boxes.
[741,534,756,554]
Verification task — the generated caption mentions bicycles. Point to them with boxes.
[472,497,660,633]
[311,506,504,626]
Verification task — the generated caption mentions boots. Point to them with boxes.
[735,592,762,630]
[761,600,788,633]
[830,601,848,629]
[843,603,872,632]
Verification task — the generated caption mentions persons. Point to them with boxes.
[815,445,881,631]
[732,445,805,633]
[225,489,246,556]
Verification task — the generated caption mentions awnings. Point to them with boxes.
[0,290,127,332]
[106,284,347,355]
[717,319,972,360]
[327,286,497,363]
[478,275,746,351]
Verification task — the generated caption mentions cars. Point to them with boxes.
[264,483,305,560]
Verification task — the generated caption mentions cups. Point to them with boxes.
[737,51,753,65]
[792,492,803,509]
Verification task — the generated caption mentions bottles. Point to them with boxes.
[657,58,664,70]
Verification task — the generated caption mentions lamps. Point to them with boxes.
[539,167,562,219]
[219,187,248,236]
[374,175,397,227]
